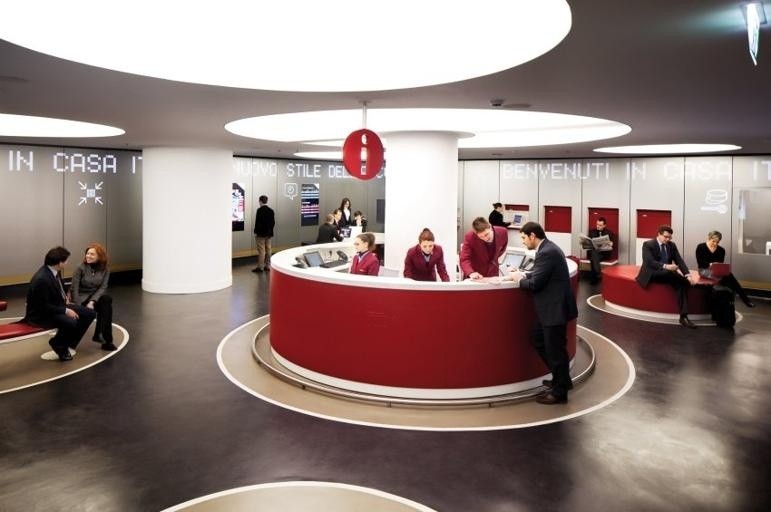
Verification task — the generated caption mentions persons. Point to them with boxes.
[460,217,508,280]
[316,198,367,243]
[489,203,512,229]
[350,233,380,275]
[504,222,579,406]
[24,247,96,361]
[696,231,756,308]
[71,243,118,351]
[251,196,275,273]
[588,217,615,286]
[404,228,450,282]
[635,224,695,329]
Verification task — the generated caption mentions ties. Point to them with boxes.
[56,274,67,304]
[660,244,667,263]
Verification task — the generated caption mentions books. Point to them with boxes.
[578,233,613,251]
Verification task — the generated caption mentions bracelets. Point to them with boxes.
[665,264,667,271]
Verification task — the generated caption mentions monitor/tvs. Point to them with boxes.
[304,251,324,267]
[501,253,526,270]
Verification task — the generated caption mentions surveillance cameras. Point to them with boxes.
[489,100,504,106]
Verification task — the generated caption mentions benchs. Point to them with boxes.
[599,264,716,321]
[0,314,61,345]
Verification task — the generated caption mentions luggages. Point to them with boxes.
[711,284,735,328]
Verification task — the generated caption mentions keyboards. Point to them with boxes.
[320,260,347,268]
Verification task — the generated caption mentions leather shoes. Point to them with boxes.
[536,392,567,404]
[101,343,116,351]
[679,316,696,329]
[92,336,105,342]
[744,300,755,308]
[543,379,573,390]
[48,338,71,360]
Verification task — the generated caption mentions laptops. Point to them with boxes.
[339,228,351,239]
[513,214,522,225]
[709,263,730,277]
